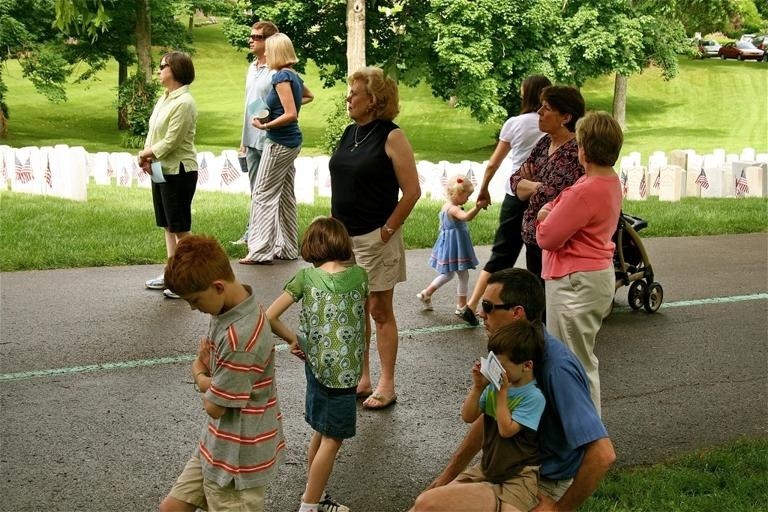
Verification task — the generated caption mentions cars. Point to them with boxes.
[690,34,767,61]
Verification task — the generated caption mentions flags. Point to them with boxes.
[1,155,240,188]
[620,167,749,198]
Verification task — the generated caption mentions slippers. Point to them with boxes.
[363,392,397,410]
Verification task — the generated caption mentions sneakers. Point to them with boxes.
[145,273,165,289]
[458,305,479,325]
[318,494,352,512]
[163,289,180,298]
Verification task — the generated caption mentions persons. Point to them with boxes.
[158,233,286,512]
[138,51,199,299]
[510,84,585,327]
[408,320,547,512]
[329,65,421,408]
[416,174,488,317]
[414,268,617,512]
[229,22,278,245]
[265,216,370,512]
[459,74,546,326]
[536,109,623,420]
[239,33,315,265]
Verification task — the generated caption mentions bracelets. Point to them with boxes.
[264,123,270,130]
[194,370,209,393]
[384,224,395,235]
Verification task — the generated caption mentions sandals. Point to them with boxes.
[455,304,479,316]
[417,289,435,311]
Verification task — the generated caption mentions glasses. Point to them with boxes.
[250,35,270,41]
[160,64,169,70]
[482,302,525,313]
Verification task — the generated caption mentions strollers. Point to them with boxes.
[603,203,663,319]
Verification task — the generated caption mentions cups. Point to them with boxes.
[254,109,270,124]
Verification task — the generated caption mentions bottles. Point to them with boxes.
[237,148,248,173]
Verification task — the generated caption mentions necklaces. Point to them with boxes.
[351,120,381,152]
